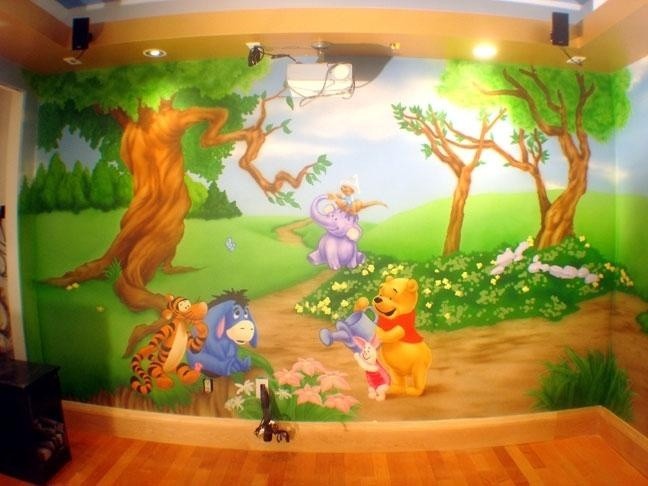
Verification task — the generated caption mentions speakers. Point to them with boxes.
[551,12,569,46]
[71,16,90,50]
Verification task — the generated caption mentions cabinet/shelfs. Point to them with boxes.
[0,359,72,486]
